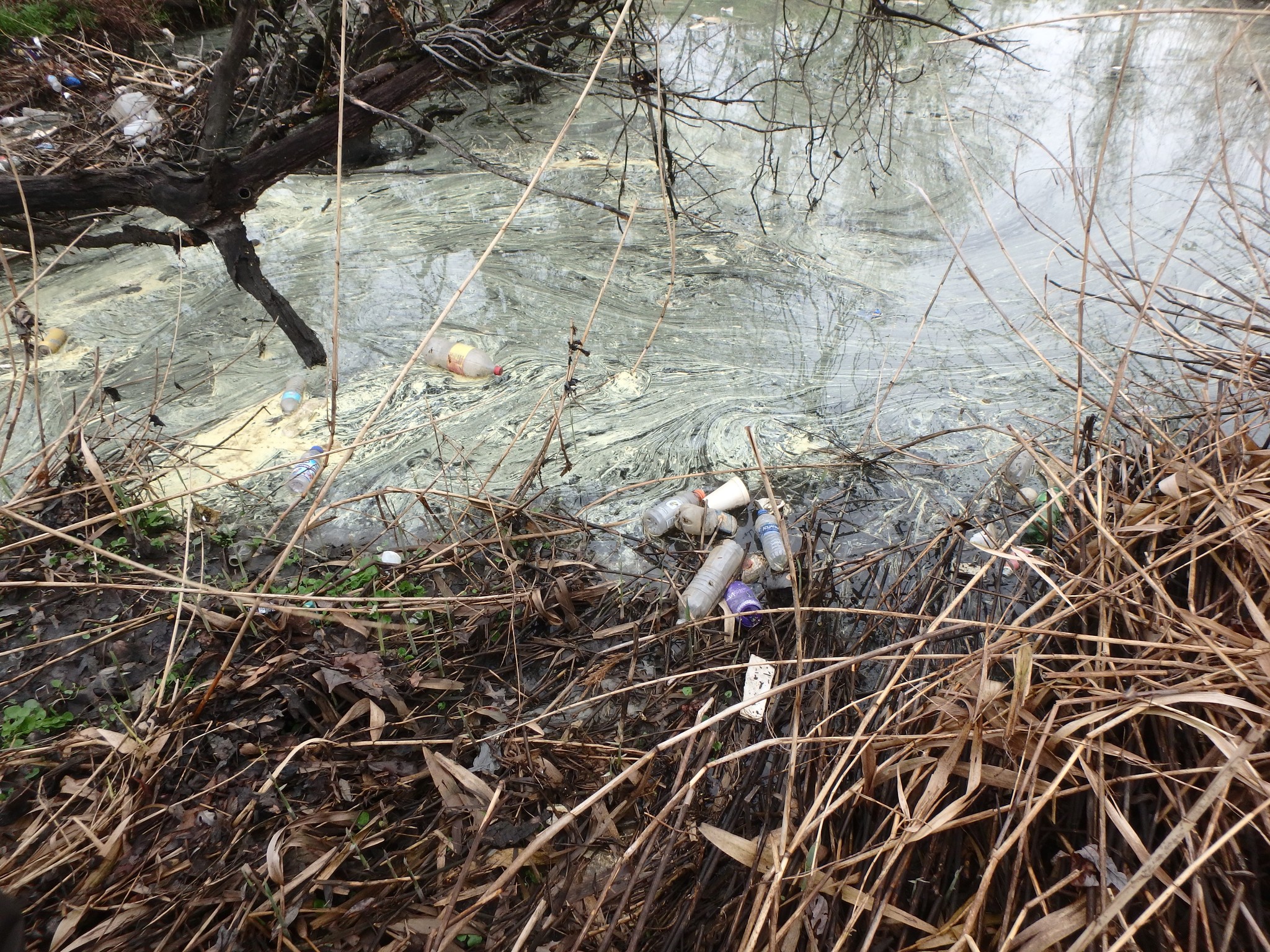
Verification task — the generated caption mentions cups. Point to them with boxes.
[702,476,750,512]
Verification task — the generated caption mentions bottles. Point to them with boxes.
[280,374,306,416]
[421,337,503,377]
[674,503,738,535]
[287,446,324,493]
[228,539,253,567]
[642,489,706,537]
[755,509,788,571]
[0,116,28,127]
[47,75,61,92]
[1026,487,1062,542]
[676,539,744,625]
